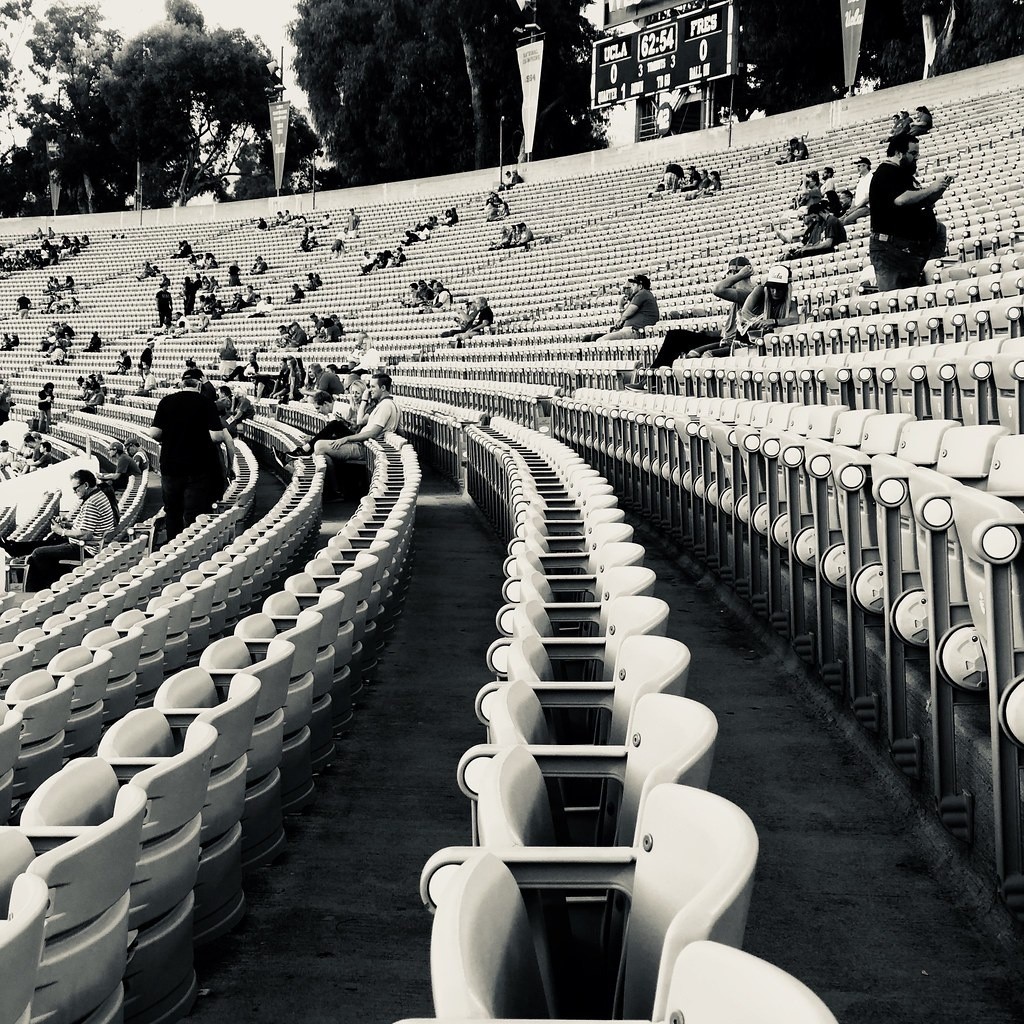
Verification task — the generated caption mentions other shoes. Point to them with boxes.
[222,374,234,382]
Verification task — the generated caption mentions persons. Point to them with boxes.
[587,105,959,393]
[1,169,534,594]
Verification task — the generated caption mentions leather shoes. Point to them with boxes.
[0,534,15,558]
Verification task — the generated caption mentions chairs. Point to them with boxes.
[0,86,1024,1024]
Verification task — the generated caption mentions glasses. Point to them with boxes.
[465,302,476,305]
[907,149,919,156]
[857,165,860,167]
[72,483,83,492]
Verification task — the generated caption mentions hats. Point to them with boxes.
[628,274,650,287]
[726,257,752,275]
[124,438,138,451]
[686,166,696,170]
[900,110,909,116]
[806,170,819,177]
[120,349,128,355]
[32,433,40,438]
[309,312,318,317]
[108,441,123,452]
[763,265,789,289]
[91,331,99,335]
[853,157,871,165]
[24,433,31,436]
[181,368,204,382]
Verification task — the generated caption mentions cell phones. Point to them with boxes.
[49,517,59,525]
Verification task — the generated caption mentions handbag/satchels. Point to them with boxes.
[144,373,157,392]
[920,222,946,260]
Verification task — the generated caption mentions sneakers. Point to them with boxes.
[324,487,345,504]
[270,445,289,469]
[624,375,647,392]
[776,230,793,242]
[285,446,311,460]
